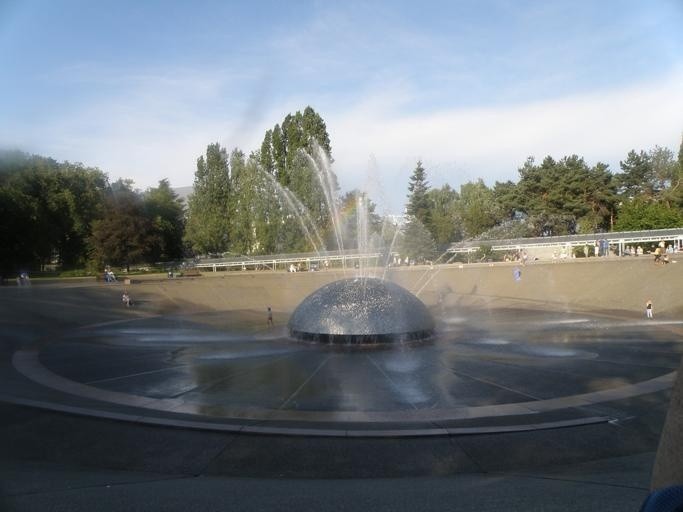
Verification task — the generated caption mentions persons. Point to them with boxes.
[512,265,521,281]
[624,240,674,264]
[266,307,274,326]
[645,299,652,319]
[122,290,134,308]
[15,272,31,287]
[583,237,608,258]
[393,256,409,267]
[642,347,683,512]
[551,250,559,264]
[104,268,119,284]
[559,249,568,264]
[519,251,529,265]
[167,271,173,279]
[289,264,297,273]
[324,260,328,270]
